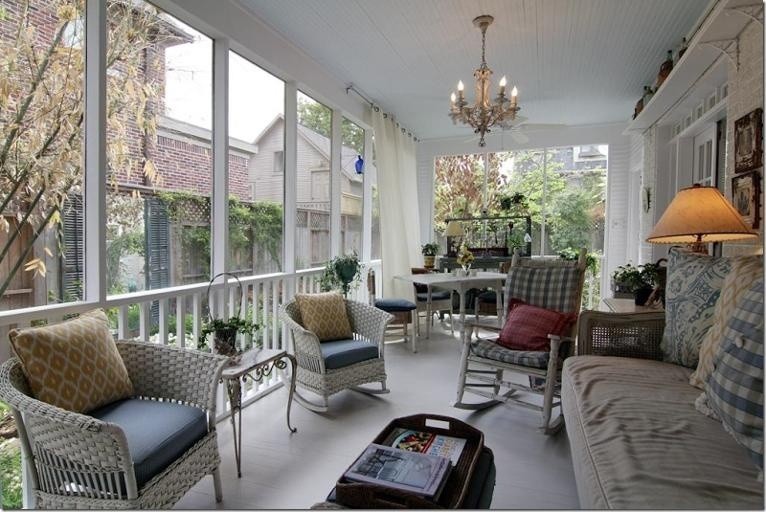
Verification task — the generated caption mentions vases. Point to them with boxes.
[462,263,472,276]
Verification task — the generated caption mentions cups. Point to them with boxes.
[452,268,478,279]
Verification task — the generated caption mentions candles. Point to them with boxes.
[499,75,507,94]
[451,91,457,110]
[458,80,465,100]
[511,86,518,106]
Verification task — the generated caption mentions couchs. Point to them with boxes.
[561,311,763,510]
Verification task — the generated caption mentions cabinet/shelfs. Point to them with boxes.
[621,0,765,146]
[440,216,532,321]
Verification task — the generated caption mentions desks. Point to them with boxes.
[393,270,508,356]
[209,345,297,477]
[599,298,665,336]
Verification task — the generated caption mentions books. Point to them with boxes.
[346,466,453,506]
[344,442,452,499]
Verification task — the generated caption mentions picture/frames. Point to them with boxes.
[734,108,763,173]
[731,172,761,230]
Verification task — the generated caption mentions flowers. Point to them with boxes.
[457,248,475,265]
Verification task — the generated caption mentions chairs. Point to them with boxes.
[0,308,231,509]
[449,247,586,436]
[367,267,418,353]
[279,290,395,413]
[475,261,510,338]
[409,268,455,339]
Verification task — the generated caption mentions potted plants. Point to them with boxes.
[625,264,658,306]
[609,260,641,299]
[196,273,259,357]
[420,242,440,268]
[314,249,364,296]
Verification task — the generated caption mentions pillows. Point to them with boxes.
[7,309,136,414]
[496,298,578,350]
[695,277,764,456]
[664,246,743,369]
[294,291,354,342]
[690,255,765,390]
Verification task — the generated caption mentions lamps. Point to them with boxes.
[448,14,522,146]
[441,219,467,256]
[644,181,758,256]
